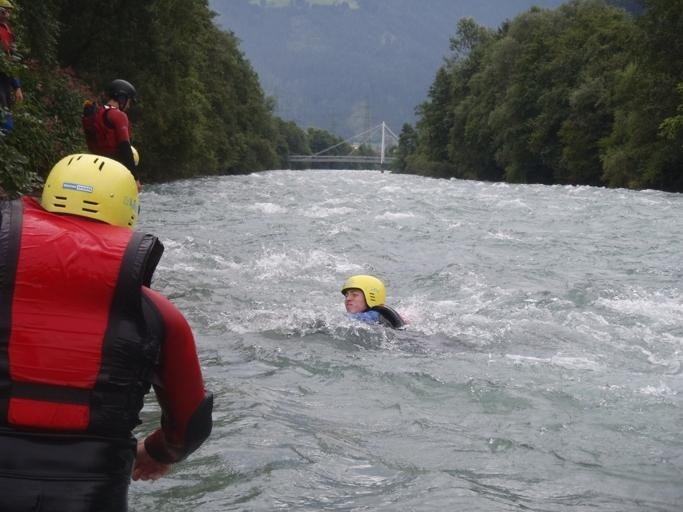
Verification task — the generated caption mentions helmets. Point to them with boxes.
[40,153,139,228]
[341,275,386,309]
[0,0,14,8]
[131,145,139,166]
[109,79,137,102]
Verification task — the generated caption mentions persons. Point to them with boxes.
[83,77,143,194]
[0,0,25,64]
[0,153,214,512]
[341,274,406,330]
[0,68,24,135]
